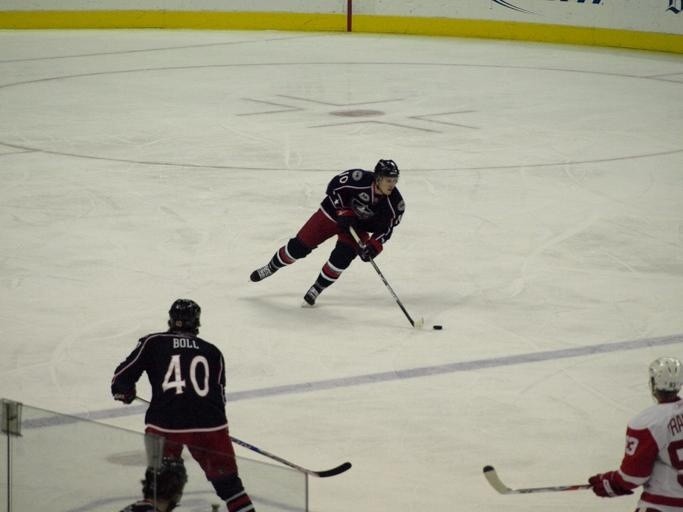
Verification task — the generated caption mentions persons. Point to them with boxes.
[249,158,406,306]
[117,454,187,512]
[109,300,255,512]
[586,357,683,512]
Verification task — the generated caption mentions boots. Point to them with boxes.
[251,262,278,282]
[304,284,324,305]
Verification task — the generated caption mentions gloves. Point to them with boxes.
[357,239,383,263]
[115,388,136,403]
[336,207,357,234]
[589,473,633,498]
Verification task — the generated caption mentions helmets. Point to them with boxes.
[168,298,201,334]
[374,159,400,179]
[648,358,682,394]
[142,457,188,500]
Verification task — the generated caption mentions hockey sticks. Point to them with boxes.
[481,464,595,495]
[134,395,352,477]
[347,224,442,331]
